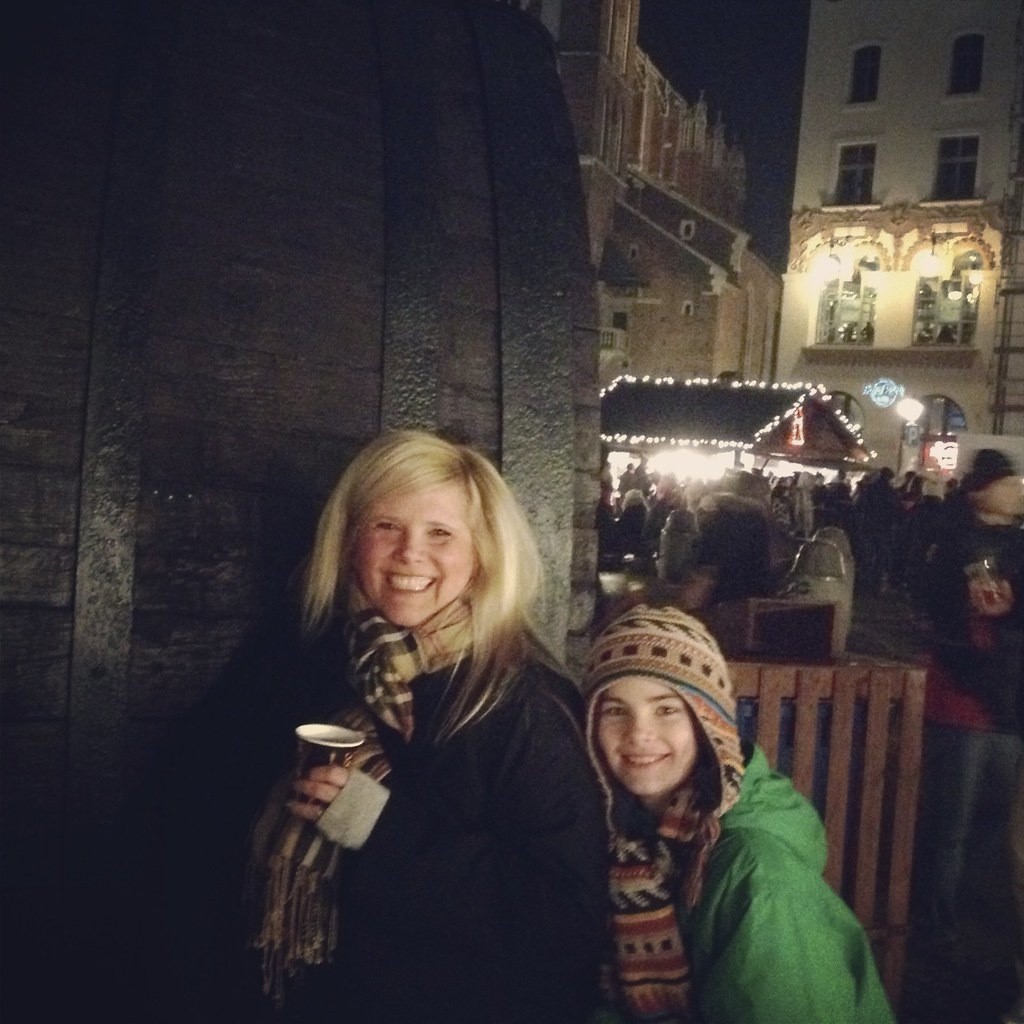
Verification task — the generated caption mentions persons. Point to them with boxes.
[595,439,1024,940]
[148,429,617,1024]
[580,605,898,1024]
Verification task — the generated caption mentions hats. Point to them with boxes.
[585,602,745,912]
[961,448,1016,491]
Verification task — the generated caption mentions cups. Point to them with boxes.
[967,555,1011,615]
[295,721,365,789]
